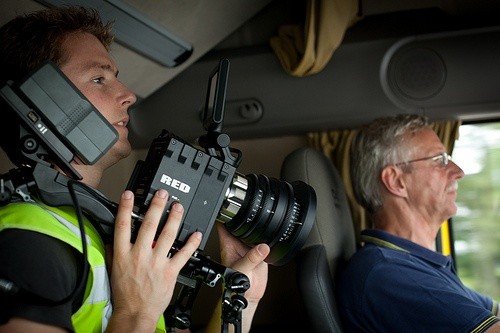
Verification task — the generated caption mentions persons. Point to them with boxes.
[342,116,500,333]
[0,5,270,333]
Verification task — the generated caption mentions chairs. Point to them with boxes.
[280,144,359,333]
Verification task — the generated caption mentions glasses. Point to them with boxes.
[379,151,452,176]
